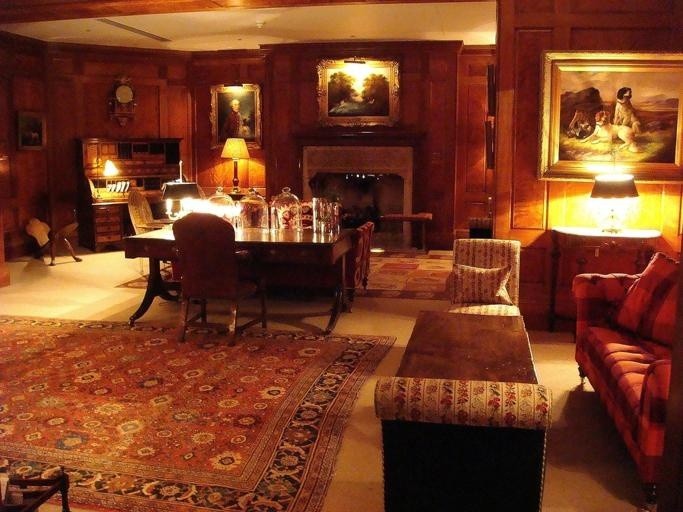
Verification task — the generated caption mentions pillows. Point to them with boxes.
[611,250,678,349]
[448,261,515,307]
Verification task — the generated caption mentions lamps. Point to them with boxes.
[220,138,251,195]
[588,173,640,236]
[87,157,119,180]
[341,56,367,67]
[220,80,244,89]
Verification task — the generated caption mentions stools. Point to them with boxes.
[1,461,70,511]
[380,212,432,254]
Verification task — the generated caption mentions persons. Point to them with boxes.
[221,100,245,142]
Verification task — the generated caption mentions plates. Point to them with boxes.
[107,181,130,193]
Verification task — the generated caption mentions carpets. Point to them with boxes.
[0,312,398,511]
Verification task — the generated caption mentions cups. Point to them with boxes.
[313,221,341,238]
[312,197,342,220]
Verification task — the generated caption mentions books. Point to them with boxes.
[105,182,129,193]
[119,143,131,159]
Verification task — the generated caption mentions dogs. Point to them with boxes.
[612,87,641,135]
[581,110,642,154]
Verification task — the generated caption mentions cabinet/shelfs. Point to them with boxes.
[73,133,186,258]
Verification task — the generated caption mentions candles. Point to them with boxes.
[488,197,492,213]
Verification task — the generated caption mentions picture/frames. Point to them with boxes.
[14,107,46,151]
[209,82,263,152]
[315,56,401,127]
[537,49,683,183]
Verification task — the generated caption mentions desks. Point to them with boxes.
[543,224,662,334]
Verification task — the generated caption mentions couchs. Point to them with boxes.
[565,264,677,504]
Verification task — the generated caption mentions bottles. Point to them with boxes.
[208,186,302,230]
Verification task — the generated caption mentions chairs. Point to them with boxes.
[172,213,266,346]
[118,182,374,335]
[373,369,555,512]
[468,217,493,240]
[447,238,521,315]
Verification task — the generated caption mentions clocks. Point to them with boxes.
[104,75,135,126]
